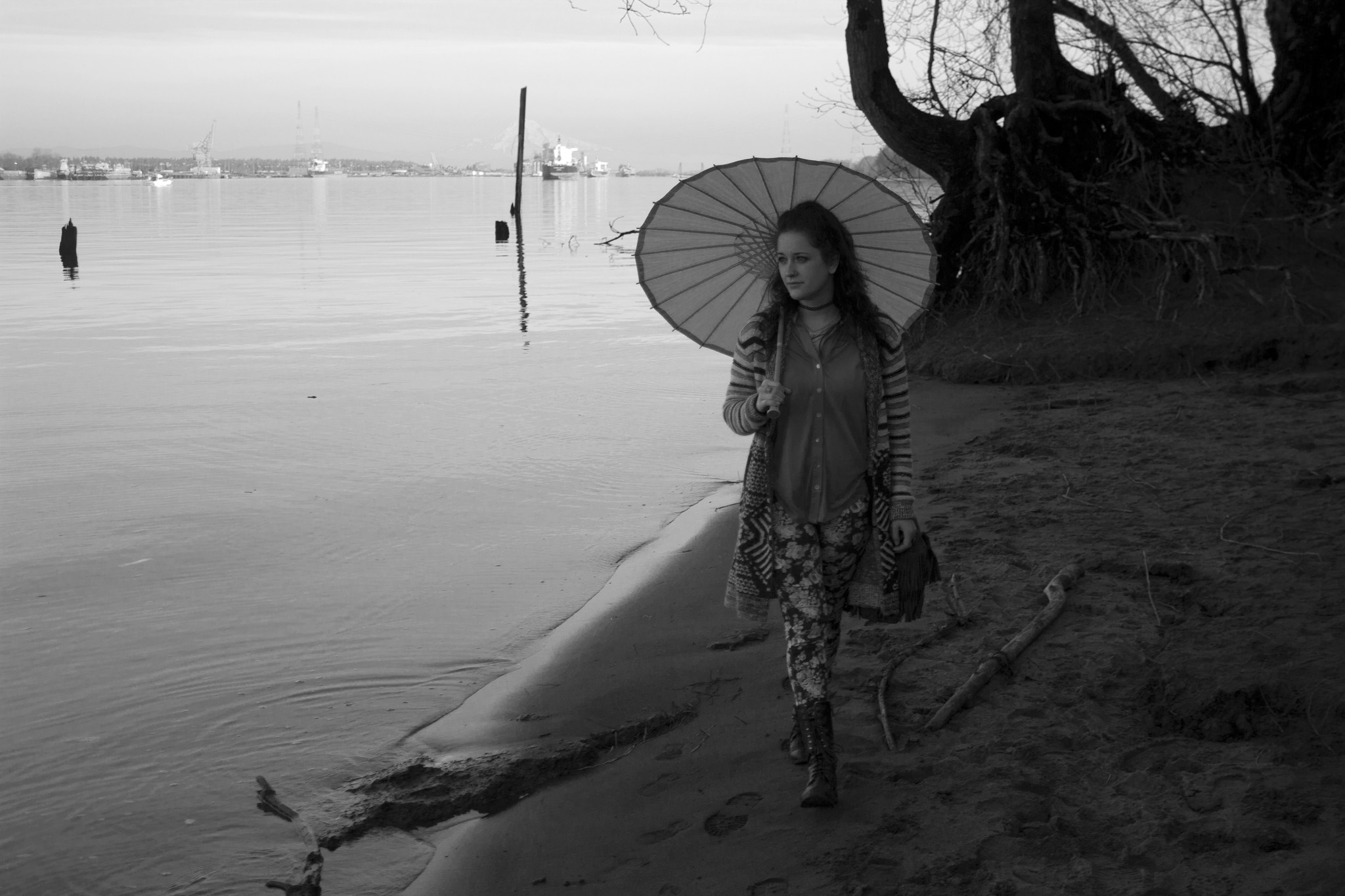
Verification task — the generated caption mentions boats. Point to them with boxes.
[103,167,131,180]
[532,133,638,181]
[153,174,174,188]
[306,156,329,179]
[0,170,28,181]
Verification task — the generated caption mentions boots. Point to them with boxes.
[790,723,807,764]
[793,701,837,808]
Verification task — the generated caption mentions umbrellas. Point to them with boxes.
[630,155,936,417]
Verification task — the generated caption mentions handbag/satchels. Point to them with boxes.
[895,530,940,622]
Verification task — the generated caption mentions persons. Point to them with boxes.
[723,200,941,811]
